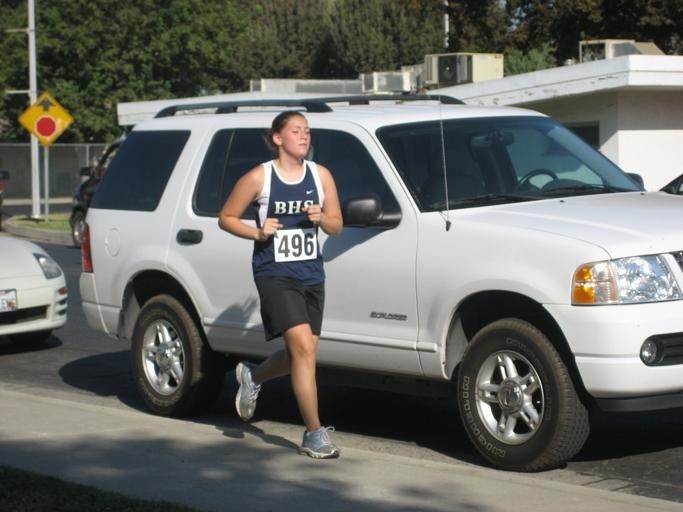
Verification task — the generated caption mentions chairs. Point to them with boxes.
[424,146,488,204]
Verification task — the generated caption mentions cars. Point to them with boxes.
[0,227,70,346]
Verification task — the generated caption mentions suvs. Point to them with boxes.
[80,102,682,474]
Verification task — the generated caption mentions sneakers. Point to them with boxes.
[298,424,340,460]
[231,359,262,422]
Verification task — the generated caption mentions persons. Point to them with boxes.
[217,110,344,460]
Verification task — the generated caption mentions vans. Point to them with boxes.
[67,93,463,249]
